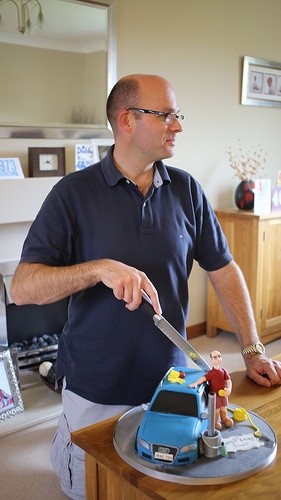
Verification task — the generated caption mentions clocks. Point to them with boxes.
[28,147,65,177]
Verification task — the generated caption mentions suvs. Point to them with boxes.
[134,366,212,466]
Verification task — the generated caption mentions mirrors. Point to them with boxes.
[0,0,118,139]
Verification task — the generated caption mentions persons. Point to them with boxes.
[267,76,274,94]
[253,75,260,90]
[10,74,281,500]
[188,351,233,429]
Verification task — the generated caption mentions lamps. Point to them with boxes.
[0,0,44,34]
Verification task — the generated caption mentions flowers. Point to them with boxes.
[227,139,267,181]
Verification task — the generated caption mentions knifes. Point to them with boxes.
[138,289,211,373]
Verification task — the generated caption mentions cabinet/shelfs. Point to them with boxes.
[206,207,281,344]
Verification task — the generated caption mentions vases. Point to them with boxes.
[234,180,255,210]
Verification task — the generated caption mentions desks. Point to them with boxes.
[69,352,281,500]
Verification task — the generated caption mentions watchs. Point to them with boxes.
[241,341,265,356]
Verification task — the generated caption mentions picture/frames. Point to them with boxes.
[0,350,24,422]
[240,56,281,108]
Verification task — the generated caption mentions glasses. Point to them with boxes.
[125,107,185,125]
